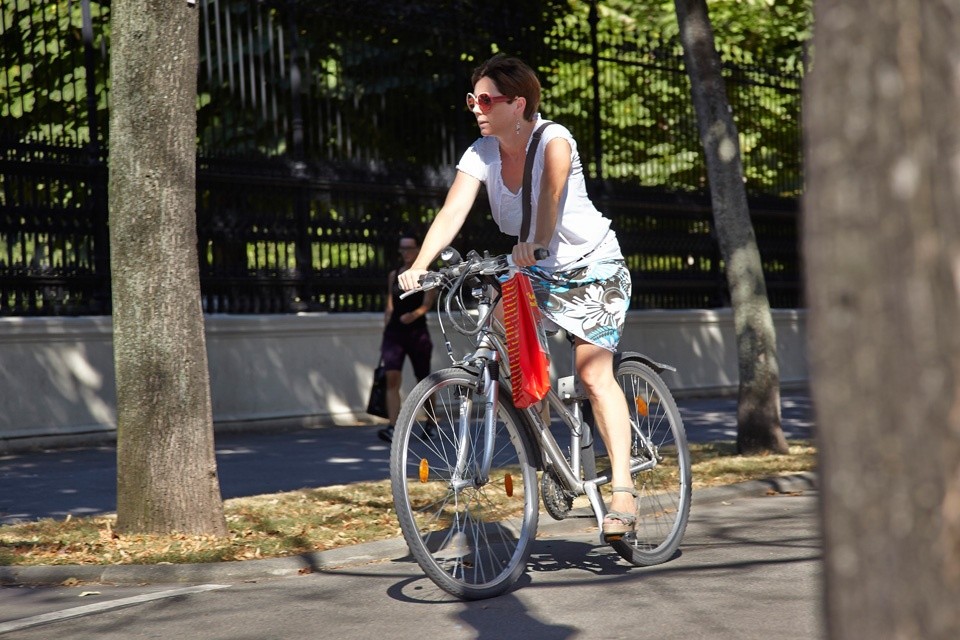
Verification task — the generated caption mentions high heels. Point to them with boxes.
[602,486,640,534]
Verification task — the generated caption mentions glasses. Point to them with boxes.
[466,92,514,112]
[397,246,417,251]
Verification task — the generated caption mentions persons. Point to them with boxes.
[377,235,439,442]
[397,57,640,535]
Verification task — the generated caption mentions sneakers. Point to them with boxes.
[423,419,437,439]
[378,425,394,443]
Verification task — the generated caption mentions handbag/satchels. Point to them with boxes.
[367,355,390,420]
[501,254,552,409]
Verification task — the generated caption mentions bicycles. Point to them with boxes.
[389,245,693,599]
